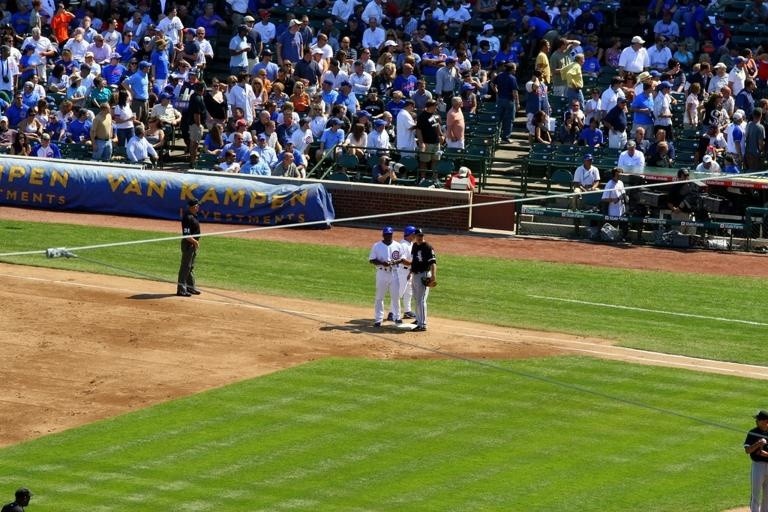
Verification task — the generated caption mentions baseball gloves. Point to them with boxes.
[422,275,437,288]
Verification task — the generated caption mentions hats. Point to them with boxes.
[752,410,768,421]
[415,228,424,236]
[383,226,393,234]
[15,487,34,497]
[404,226,416,235]
[0,35,198,141]
[584,37,768,175]
[226,0,518,160]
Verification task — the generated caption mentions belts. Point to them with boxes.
[404,266,408,269]
[412,270,429,275]
[378,267,392,272]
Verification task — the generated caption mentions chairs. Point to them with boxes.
[421,74,437,93]
[164,124,172,138]
[581,72,601,100]
[440,112,447,126]
[195,153,218,170]
[548,96,567,114]
[271,7,334,30]
[465,102,502,194]
[723,3,765,50]
[58,144,88,160]
[676,129,700,167]
[670,94,687,127]
[325,149,461,187]
[520,144,619,193]
[145,137,162,156]
[47,93,66,104]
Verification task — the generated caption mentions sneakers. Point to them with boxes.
[404,311,416,319]
[186,287,201,295]
[387,312,403,324]
[374,321,381,329]
[410,326,427,332]
[410,320,419,325]
[176,290,192,297]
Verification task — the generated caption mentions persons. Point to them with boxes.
[368,226,406,327]
[406,227,437,331]
[387,225,417,322]
[743,410,768,512]
[1,487,33,512]
[176,198,202,297]
